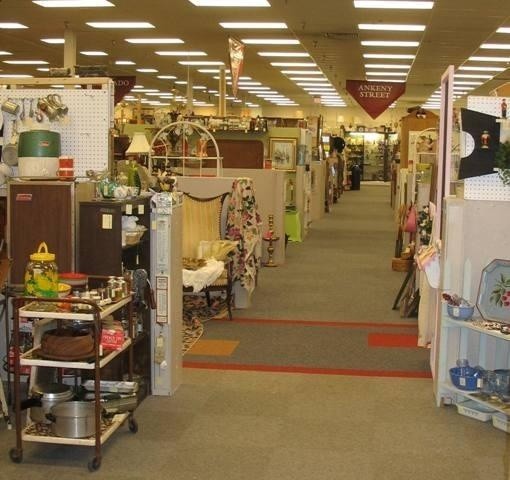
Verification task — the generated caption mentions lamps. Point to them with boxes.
[124,131,154,164]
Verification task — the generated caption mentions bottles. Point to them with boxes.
[116,276,128,297]
[105,276,118,298]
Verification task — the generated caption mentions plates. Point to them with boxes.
[476,258,510,324]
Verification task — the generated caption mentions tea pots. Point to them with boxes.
[36,92,67,124]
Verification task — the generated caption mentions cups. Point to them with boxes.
[471,366,484,378]
[456,359,469,377]
[1,100,20,115]
[58,155,75,178]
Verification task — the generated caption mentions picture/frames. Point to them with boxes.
[269,138,296,173]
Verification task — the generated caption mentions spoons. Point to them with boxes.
[19,100,26,120]
[36,109,44,124]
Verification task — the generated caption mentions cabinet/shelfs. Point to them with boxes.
[79,195,152,405]
[437,296,510,420]
[321,129,398,182]
[405,125,438,208]
[8,274,139,472]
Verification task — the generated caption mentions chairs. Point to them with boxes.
[175,193,234,318]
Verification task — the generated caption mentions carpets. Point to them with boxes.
[181,318,435,378]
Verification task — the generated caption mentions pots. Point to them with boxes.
[46,400,106,439]
[14,383,75,425]
[2,120,20,166]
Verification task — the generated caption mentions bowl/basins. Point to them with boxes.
[447,303,475,322]
[36,328,97,360]
[57,273,87,290]
[58,284,70,297]
[449,367,484,390]
[126,227,148,245]
